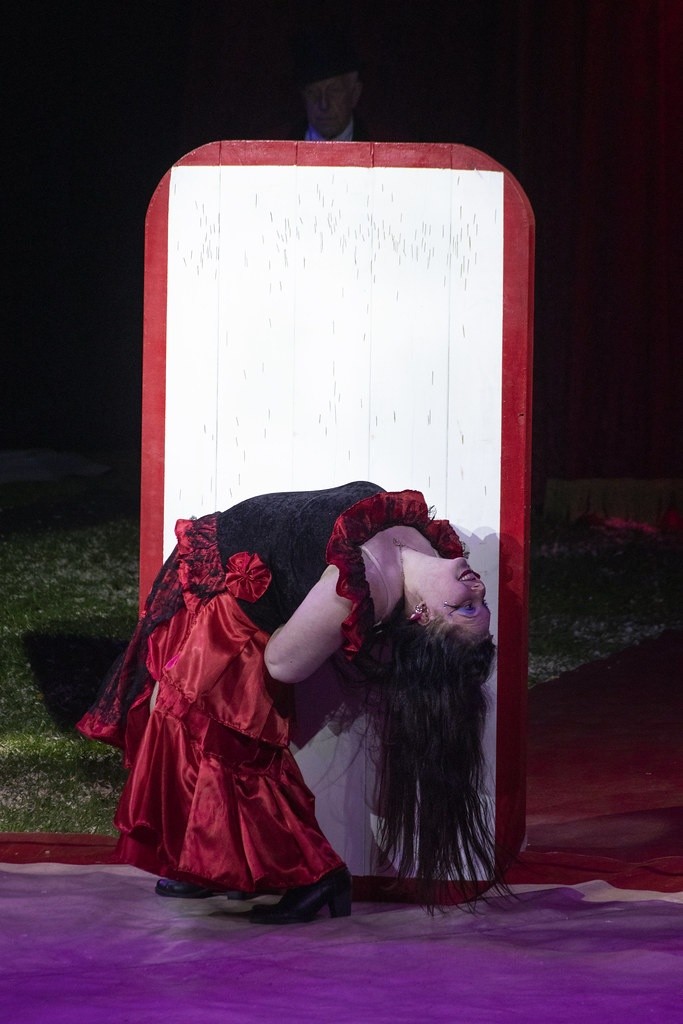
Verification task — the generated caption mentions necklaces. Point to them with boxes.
[392,537,419,581]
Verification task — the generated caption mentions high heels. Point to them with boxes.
[155,880,253,901]
[249,867,353,925]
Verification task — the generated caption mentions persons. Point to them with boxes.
[75,482,524,925]
